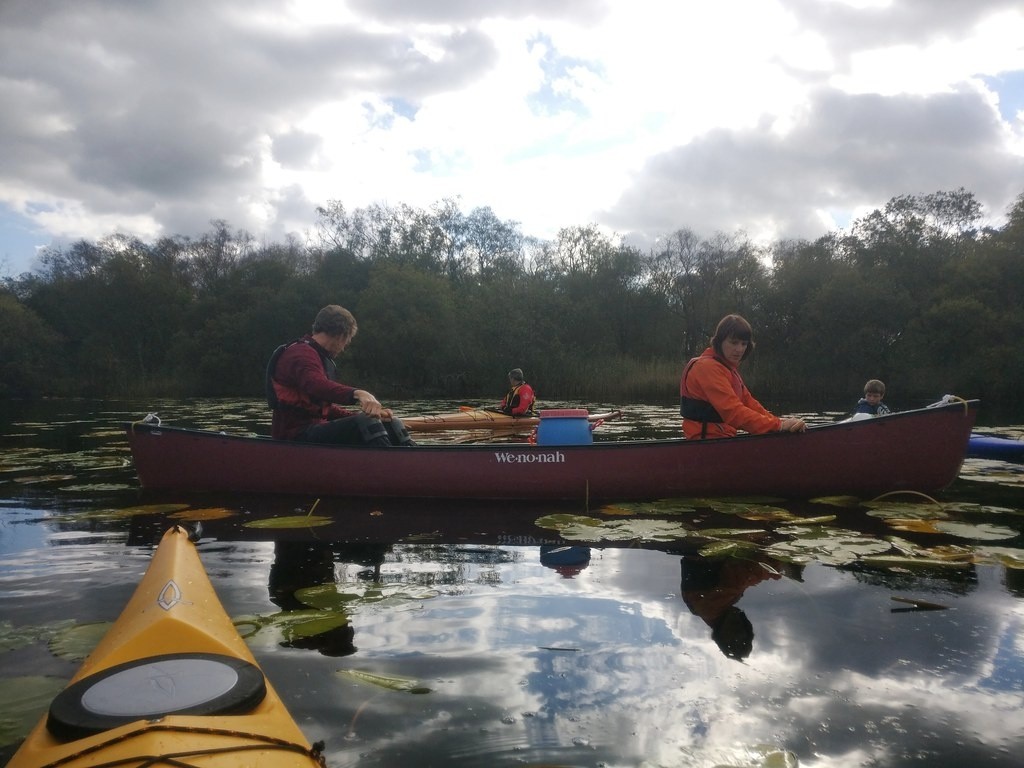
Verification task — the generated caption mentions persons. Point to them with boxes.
[680,552,782,660]
[850,380,891,420]
[498,368,535,416]
[266,532,359,657]
[272,304,419,446]
[680,314,808,439]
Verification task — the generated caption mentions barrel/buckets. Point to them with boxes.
[537,409,593,444]
[540,546,590,576]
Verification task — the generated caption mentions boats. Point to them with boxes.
[397,406,624,431]
[966,433,1024,464]
[2,519,326,768]
[125,398,980,504]
